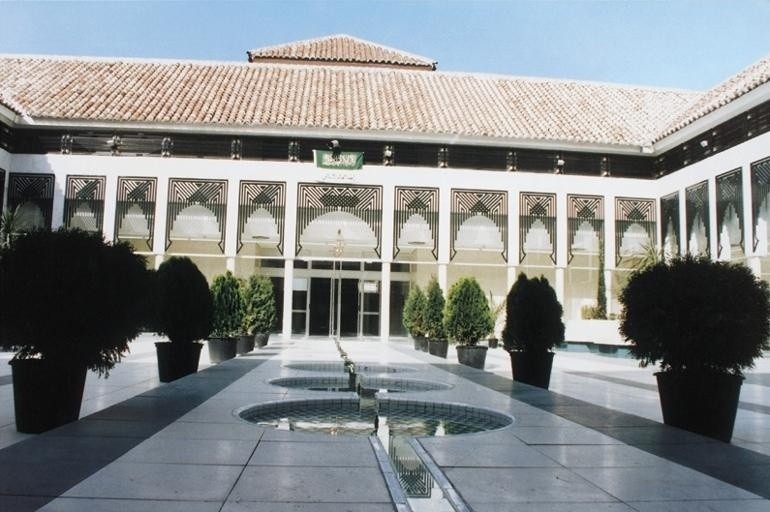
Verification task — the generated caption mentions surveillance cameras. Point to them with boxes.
[326,139,341,150]
